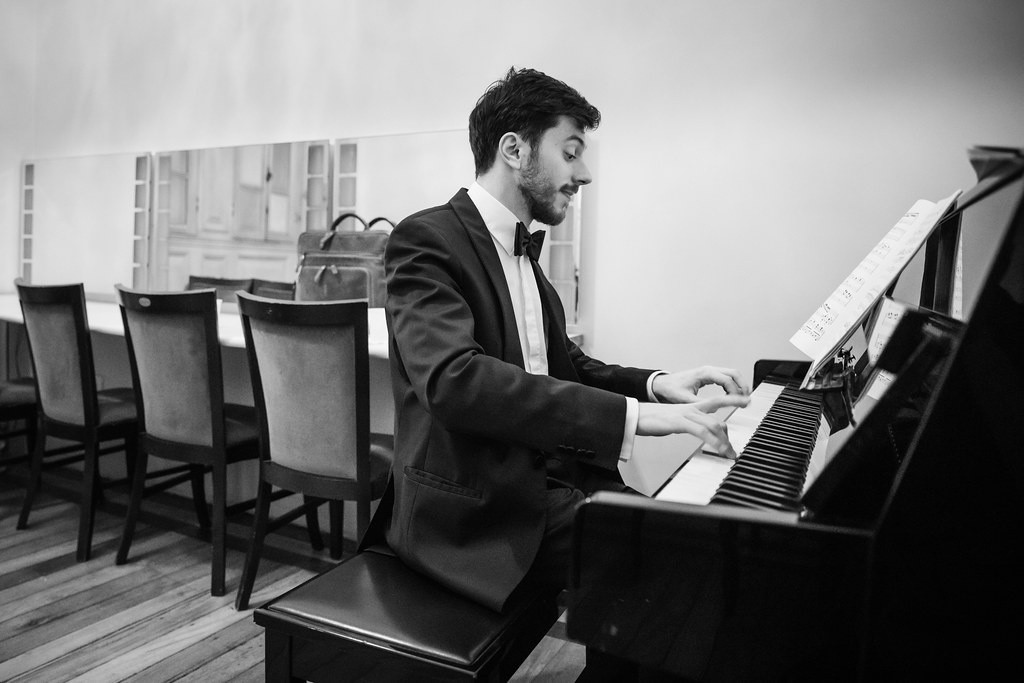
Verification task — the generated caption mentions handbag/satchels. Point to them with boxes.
[295,213,397,308]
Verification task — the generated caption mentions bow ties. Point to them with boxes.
[513,221,547,263]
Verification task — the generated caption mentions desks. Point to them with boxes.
[0,298,586,548]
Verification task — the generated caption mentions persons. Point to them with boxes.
[356,65,754,612]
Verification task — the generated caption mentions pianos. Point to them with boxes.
[566,149,1024,683]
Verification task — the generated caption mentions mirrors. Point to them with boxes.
[151,139,330,316]
[17,152,151,305]
[334,130,580,326]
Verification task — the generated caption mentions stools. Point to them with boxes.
[253,545,567,683]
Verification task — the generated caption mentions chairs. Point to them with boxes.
[0,377,43,499]
[186,275,252,303]
[14,283,393,614]
[250,280,296,301]
